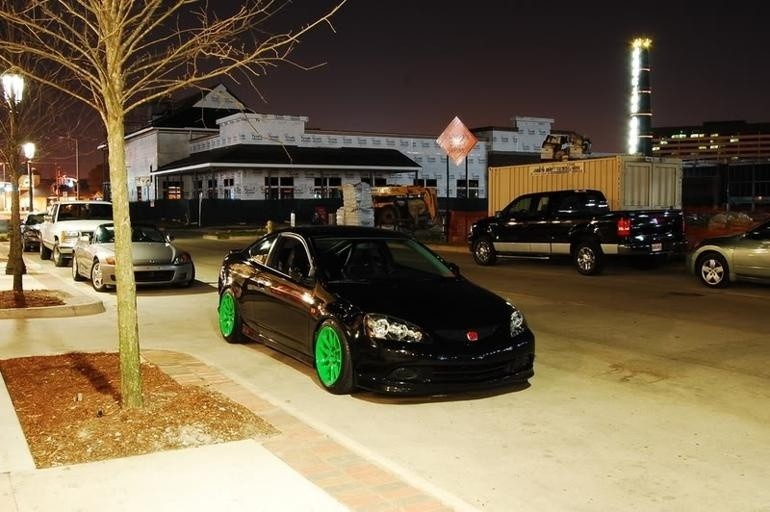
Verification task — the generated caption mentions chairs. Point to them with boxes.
[275,243,393,283]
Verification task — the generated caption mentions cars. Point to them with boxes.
[218,225,533,400]
[681,205,770,251]
[691,225,770,288]
[0,199,195,293]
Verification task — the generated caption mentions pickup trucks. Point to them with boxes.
[466,189,689,273]
